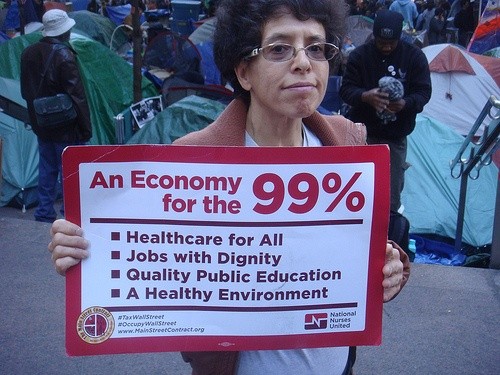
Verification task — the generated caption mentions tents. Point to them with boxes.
[0,11,499,248]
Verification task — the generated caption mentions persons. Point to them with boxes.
[21,9,93,223]
[339,9,432,214]
[48,1,411,375]
[389,0,418,33]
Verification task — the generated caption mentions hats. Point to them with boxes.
[374,10,404,45]
[39,7,77,38]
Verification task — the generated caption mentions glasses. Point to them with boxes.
[243,41,341,64]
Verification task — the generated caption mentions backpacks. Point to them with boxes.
[29,94,82,128]
